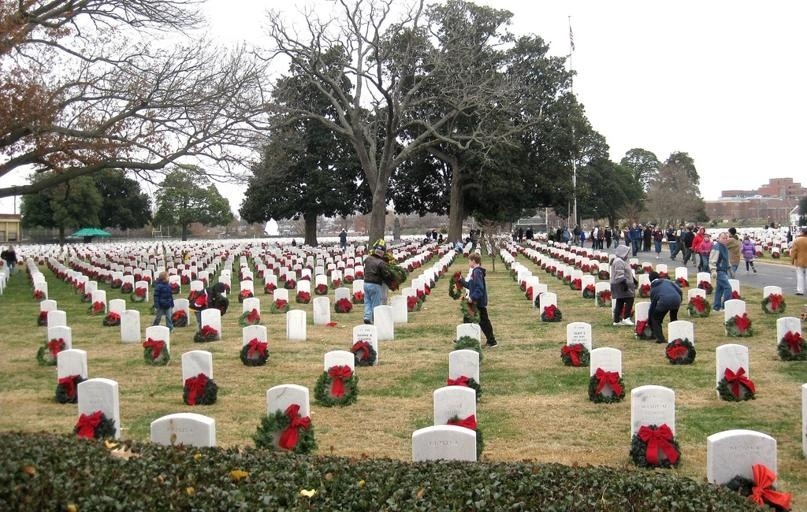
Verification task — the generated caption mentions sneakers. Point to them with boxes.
[364,319,371,324]
[620,317,635,326]
[481,339,498,350]
[612,321,623,325]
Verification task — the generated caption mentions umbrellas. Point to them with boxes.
[71,227,111,238]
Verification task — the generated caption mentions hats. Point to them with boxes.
[376,245,385,251]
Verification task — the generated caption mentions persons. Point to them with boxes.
[548,224,585,248]
[425,229,442,244]
[291,238,296,247]
[189,283,230,329]
[151,271,174,335]
[338,228,347,251]
[789,226,807,296]
[786,225,798,251]
[1,246,16,276]
[590,224,758,344]
[362,244,392,325]
[458,253,498,349]
[462,229,485,248]
[512,224,534,244]
